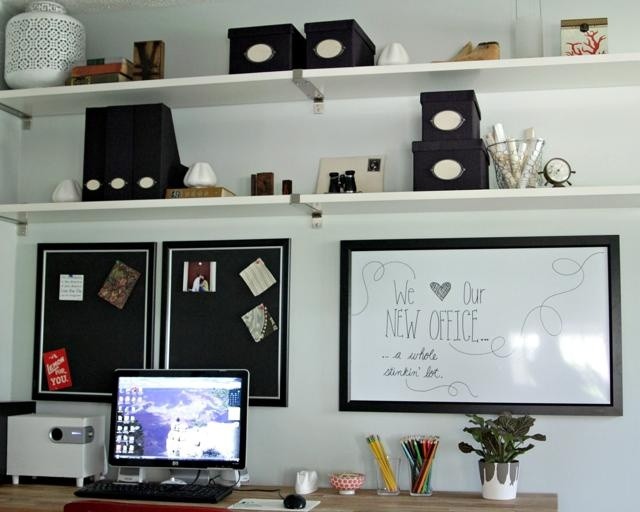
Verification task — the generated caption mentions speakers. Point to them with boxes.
[117,466,144,483]
[221,467,250,483]
[6,413,105,487]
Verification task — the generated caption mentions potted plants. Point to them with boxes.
[455,402,549,500]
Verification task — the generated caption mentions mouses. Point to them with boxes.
[283,494,306,509]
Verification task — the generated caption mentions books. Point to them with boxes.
[86,57,133,68]
[72,63,134,79]
[64,73,132,85]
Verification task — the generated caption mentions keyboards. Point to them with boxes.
[74,480,234,504]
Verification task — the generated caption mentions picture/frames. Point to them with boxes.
[28,239,159,405]
[157,236,292,411]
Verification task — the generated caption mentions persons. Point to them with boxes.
[191,270,202,292]
[198,275,209,292]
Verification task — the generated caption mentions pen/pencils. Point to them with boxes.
[366,434,439,494]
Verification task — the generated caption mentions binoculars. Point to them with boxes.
[328,171,356,193]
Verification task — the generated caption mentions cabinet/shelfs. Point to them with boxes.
[1,53,640,228]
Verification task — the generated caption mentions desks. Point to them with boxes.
[0,475,564,512]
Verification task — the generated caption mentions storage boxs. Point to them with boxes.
[415,87,484,141]
[225,20,304,72]
[409,137,494,192]
[303,15,376,69]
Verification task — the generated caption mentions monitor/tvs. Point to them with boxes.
[108,369,250,489]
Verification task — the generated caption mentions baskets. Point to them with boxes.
[486,138,545,190]
